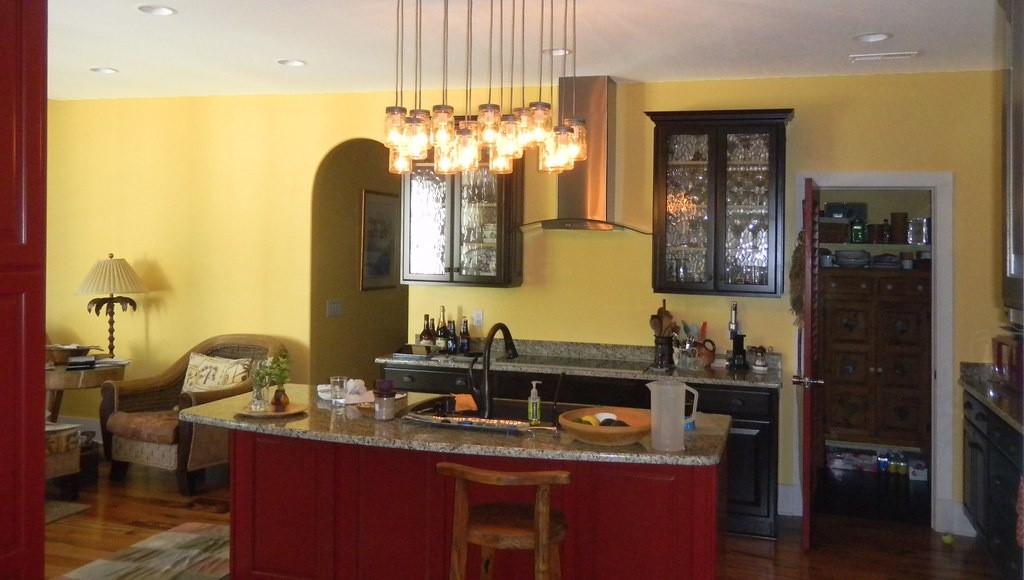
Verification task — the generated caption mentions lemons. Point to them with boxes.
[580,414,600,426]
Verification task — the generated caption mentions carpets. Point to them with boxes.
[53,521,229,580]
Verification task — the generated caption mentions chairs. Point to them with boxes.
[436,461,571,580]
[100,334,287,496]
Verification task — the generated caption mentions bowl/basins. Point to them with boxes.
[558,407,650,446]
[483,224,497,238]
[836,250,870,266]
[51,346,90,365]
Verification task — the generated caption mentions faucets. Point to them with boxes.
[468,322,519,419]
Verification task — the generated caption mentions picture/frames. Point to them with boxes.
[360,189,400,289]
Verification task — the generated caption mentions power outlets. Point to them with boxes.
[326,299,345,316]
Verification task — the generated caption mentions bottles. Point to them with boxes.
[420,306,470,355]
[883,219,892,244]
[851,219,863,243]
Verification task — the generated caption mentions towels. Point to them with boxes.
[450,393,478,412]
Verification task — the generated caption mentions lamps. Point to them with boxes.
[76,253,147,358]
[386,0,589,176]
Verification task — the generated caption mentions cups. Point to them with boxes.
[331,376,347,407]
[374,391,396,420]
[654,334,675,368]
[907,217,927,244]
[891,212,908,245]
[902,259,912,268]
[820,255,839,267]
[867,223,883,244]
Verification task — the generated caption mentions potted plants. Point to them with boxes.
[245,353,289,411]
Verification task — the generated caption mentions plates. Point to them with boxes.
[871,263,901,268]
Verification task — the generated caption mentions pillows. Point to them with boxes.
[174,351,251,410]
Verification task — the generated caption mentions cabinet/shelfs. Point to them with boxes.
[382,364,778,541]
[646,109,793,298]
[401,115,524,288]
[963,390,1024,580]
[821,268,930,448]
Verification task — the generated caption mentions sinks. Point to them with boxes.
[397,394,592,430]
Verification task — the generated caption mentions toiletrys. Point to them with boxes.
[527,380,543,425]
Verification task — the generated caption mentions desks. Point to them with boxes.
[45,359,128,423]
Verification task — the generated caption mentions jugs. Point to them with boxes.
[646,380,698,452]
[673,345,699,367]
